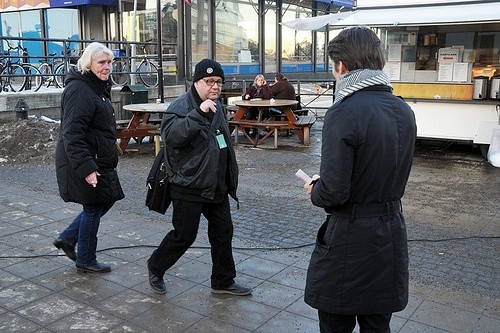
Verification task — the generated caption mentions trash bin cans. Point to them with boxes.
[118,84,149,127]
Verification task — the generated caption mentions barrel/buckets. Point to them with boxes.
[472,76,489,99]
[489,76,500,99]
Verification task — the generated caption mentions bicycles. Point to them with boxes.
[110,39,159,88]
[9,45,43,93]
[36,37,79,90]
[0,39,26,92]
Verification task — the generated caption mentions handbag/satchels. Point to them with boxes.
[145,146,171,215]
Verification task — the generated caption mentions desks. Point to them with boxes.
[119,103,171,155]
[228,98,304,145]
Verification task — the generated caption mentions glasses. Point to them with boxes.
[201,77,223,87]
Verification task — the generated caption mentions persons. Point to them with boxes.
[270,73,297,110]
[242,74,272,119]
[52,42,125,273]
[304,26,418,333]
[147,59,253,296]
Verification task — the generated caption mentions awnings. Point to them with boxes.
[326,1,500,28]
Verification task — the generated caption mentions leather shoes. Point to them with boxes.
[76,260,111,274]
[52,234,77,262]
[211,283,251,295]
[147,259,167,294]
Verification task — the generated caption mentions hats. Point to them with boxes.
[192,58,225,84]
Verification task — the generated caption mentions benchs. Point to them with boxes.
[226,106,316,149]
[116,118,164,157]
